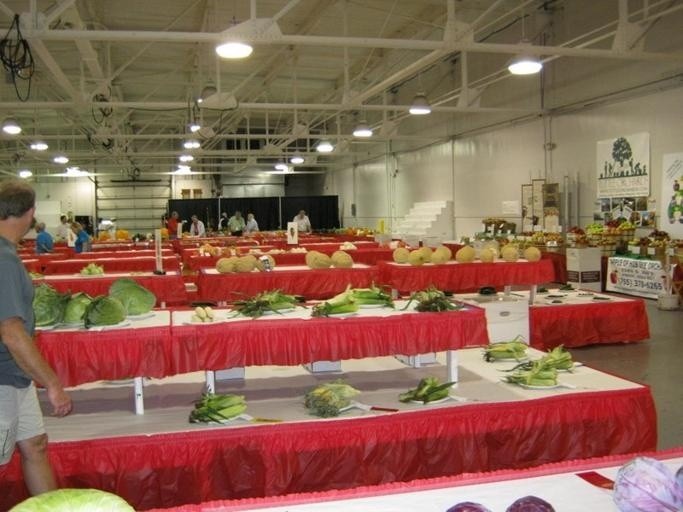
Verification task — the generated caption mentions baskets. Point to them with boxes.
[655,272,679,310]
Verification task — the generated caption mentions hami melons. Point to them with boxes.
[216,255,276,273]
[393,246,541,265]
[305,250,353,269]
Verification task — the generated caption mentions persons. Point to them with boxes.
[226,209,245,234]
[242,211,259,232]
[213,209,226,231]
[56,213,118,253]
[0,180,73,499]
[292,209,312,236]
[189,213,205,238]
[167,212,178,239]
[30,222,55,256]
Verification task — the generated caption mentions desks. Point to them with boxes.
[0,342,659,512]
[451,285,645,353]
[162,444,683,512]
[1,231,683,311]
[0,290,485,414]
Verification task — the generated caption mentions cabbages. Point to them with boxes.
[32,279,156,329]
[8,488,137,512]
[611,455,683,512]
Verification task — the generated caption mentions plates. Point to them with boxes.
[492,354,583,390]
[190,412,253,426]
[358,304,389,309]
[335,400,373,412]
[409,395,466,404]
[326,309,358,319]
[252,307,294,316]
[34,308,158,333]
[547,288,610,305]
[184,316,225,325]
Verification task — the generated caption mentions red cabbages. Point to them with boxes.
[505,495,555,512]
[446,501,492,512]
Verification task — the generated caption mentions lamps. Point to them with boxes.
[176,0,253,176]
[0,112,70,180]
[316,112,333,152]
[352,100,373,138]
[409,70,431,115]
[506,4,543,76]
[289,137,304,164]
[274,158,285,170]
